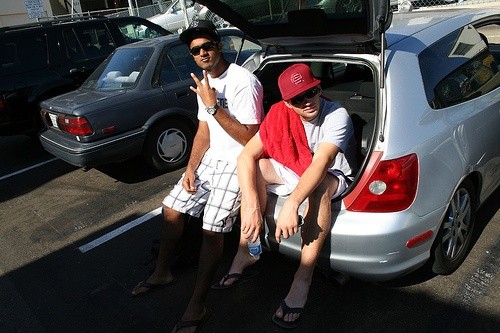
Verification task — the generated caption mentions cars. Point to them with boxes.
[35,28,263,175]
[184,0,500,283]
[133,0,232,41]
[0,14,174,189]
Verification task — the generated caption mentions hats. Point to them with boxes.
[278,63,319,100]
[179,27,221,45]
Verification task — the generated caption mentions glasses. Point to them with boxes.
[286,85,319,107]
[189,41,216,56]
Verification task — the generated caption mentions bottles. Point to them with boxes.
[248,231,263,260]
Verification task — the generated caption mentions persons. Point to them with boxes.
[211,63,354,329]
[129,20,265,333]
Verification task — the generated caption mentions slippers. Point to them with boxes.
[217,256,263,289]
[272,298,309,328]
[175,308,210,333]
[131,275,176,297]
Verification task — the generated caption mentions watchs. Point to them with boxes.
[205,104,219,116]
